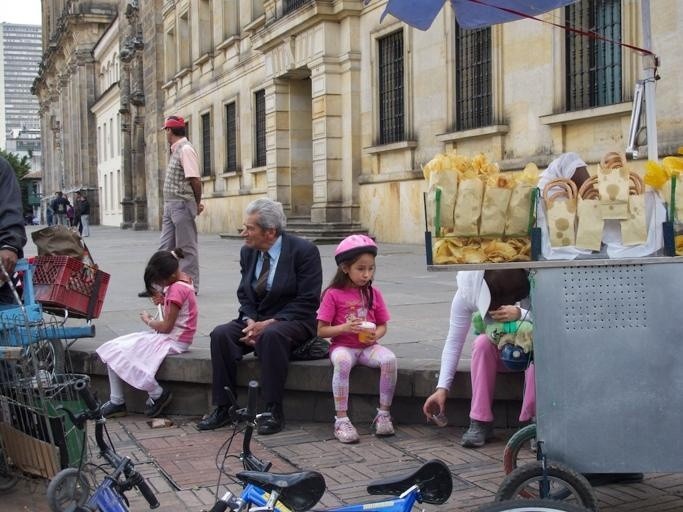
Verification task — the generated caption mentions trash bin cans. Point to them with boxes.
[9,373,91,469]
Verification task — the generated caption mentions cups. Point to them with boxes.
[427,407,451,430]
[356,320,375,344]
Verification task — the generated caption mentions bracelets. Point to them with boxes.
[146,318,154,329]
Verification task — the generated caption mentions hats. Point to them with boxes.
[30,224,85,259]
[456,270,494,320]
[160,115,185,130]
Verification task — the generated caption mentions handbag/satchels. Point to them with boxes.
[291,336,332,361]
[500,343,529,373]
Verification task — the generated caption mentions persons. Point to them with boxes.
[137,114,203,297]
[0,156,28,305]
[422,269,537,449]
[47,191,91,238]
[196,197,323,436]
[95,246,198,419]
[315,233,398,445]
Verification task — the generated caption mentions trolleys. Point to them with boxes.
[411,185,681,508]
[1,261,95,512]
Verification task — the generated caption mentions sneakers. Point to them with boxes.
[459,420,494,448]
[333,419,361,444]
[375,412,395,436]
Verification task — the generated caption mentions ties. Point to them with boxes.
[254,251,272,306]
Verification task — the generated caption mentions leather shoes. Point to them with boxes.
[197,403,237,431]
[100,401,127,419]
[139,291,151,297]
[257,412,286,435]
[144,387,173,418]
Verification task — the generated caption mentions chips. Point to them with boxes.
[421,151,541,263]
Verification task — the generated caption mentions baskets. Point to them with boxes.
[20,243,111,319]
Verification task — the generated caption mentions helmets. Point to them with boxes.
[334,233,378,266]
